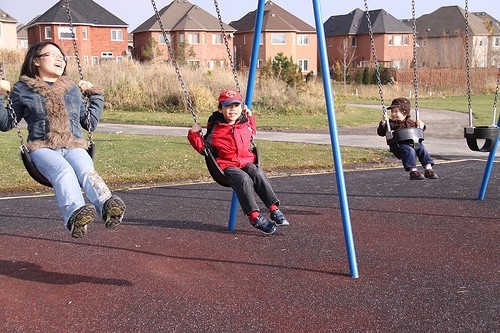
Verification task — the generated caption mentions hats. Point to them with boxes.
[218,89,243,107]
[387,98,411,115]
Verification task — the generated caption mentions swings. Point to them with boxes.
[363,0,424,158]
[463,0,500,152]
[151,0,260,188]
[0,0,95,186]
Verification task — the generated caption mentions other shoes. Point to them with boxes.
[424,169,439,179]
[410,171,424,180]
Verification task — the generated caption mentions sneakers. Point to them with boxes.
[67,203,97,238]
[270,209,290,226]
[249,212,277,234]
[101,195,126,230]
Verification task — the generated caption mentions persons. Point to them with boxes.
[377,97,439,180]
[187,89,289,234]
[0,41,127,238]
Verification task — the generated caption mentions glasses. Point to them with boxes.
[36,52,68,62]
